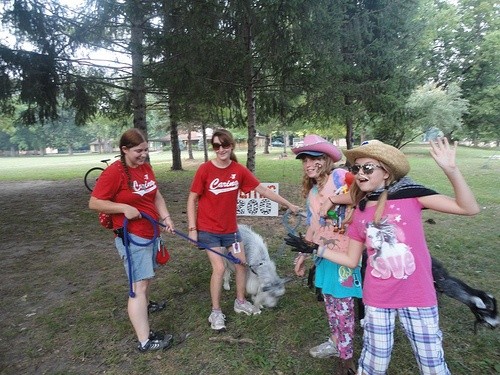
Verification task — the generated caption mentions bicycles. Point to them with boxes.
[84,154,121,193]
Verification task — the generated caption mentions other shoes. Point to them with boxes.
[148,302,164,312]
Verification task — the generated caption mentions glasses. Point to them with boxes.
[212,143,232,150]
[349,164,383,175]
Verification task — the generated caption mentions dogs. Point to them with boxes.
[220,223,286,309]
[431,255,500,334]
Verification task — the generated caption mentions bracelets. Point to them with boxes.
[188,226,197,231]
[328,196,334,204]
[162,216,170,221]
[319,246,326,257]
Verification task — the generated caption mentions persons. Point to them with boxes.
[187,128,300,330]
[293,134,363,375]
[283,137,480,375]
[88,128,175,352]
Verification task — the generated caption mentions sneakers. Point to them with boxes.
[234,298,262,315]
[138,340,170,353]
[310,342,339,360]
[149,331,172,342]
[208,311,227,331]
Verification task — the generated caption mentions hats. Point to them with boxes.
[296,151,324,159]
[342,140,410,179]
[291,134,341,163]
